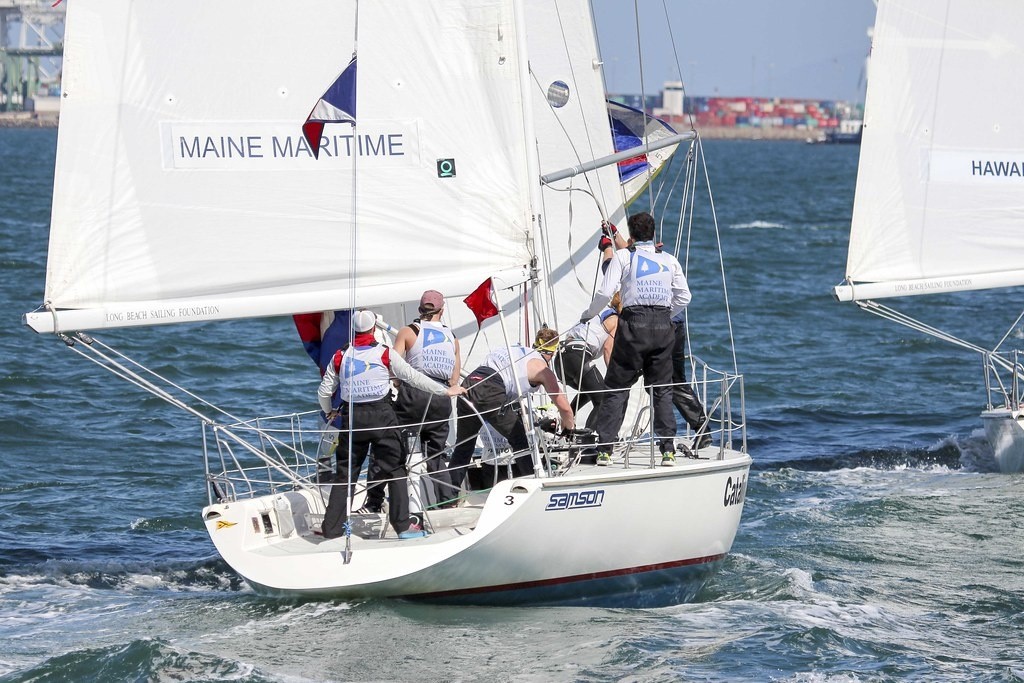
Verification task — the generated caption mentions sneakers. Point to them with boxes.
[660,450,676,464]
[596,453,613,466]
[691,428,713,451]
[398,523,428,539]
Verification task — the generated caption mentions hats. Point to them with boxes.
[351,310,375,332]
[420,290,446,313]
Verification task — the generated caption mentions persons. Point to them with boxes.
[316,309,468,540]
[596,219,713,450]
[579,211,692,465]
[551,290,622,429]
[356,290,461,514]
[448,328,575,491]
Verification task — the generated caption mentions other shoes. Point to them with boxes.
[355,504,381,514]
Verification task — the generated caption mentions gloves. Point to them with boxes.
[598,235,612,252]
[601,220,619,238]
[560,424,576,442]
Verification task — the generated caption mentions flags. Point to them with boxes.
[463,278,499,331]
[301,56,356,160]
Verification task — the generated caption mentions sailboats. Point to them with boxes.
[831,0,1024,457]
[21,0,756,603]
